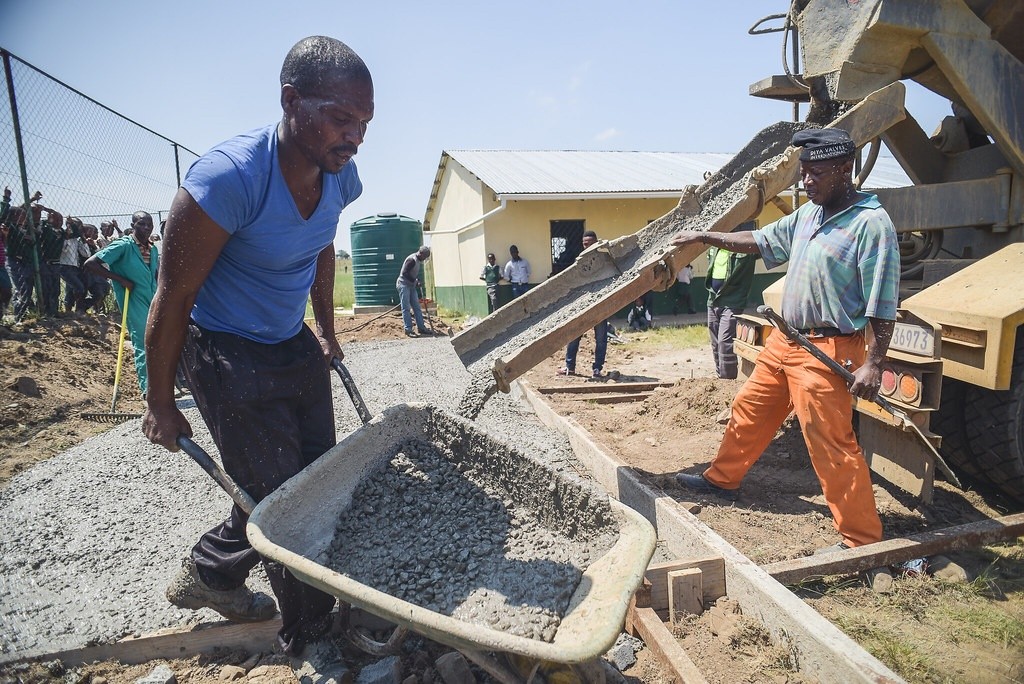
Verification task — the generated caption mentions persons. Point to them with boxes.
[395,247,432,337]
[479,253,504,314]
[557,230,608,378]
[673,263,697,314]
[142,35,373,659]
[670,128,900,555]
[504,245,532,300]
[0,185,134,315]
[84,210,192,398]
[627,298,657,331]
[705,223,763,378]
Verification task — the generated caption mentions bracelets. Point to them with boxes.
[703,231,706,245]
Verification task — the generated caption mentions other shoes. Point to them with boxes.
[405,332,419,338]
[418,329,433,334]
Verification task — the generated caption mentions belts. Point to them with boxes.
[512,283,528,286]
[794,326,855,336]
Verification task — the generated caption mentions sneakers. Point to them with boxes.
[675,472,740,500]
[291,637,352,684]
[166,557,277,621]
[593,369,601,379]
[557,367,575,373]
[815,543,850,554]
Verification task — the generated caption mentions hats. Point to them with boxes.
[420,246,430,256]
[510,245,519,253]
[792,129,857,161]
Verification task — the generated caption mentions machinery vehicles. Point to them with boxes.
[448,0,1024,511]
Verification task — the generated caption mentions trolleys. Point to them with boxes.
[178,355,658,684]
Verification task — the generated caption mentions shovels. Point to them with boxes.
[757,305,962,489]
